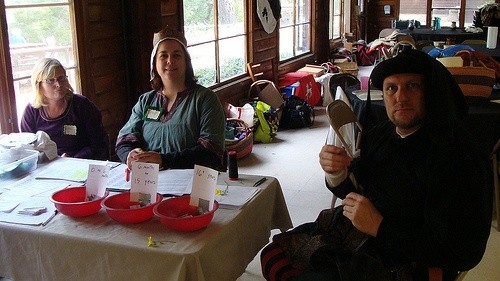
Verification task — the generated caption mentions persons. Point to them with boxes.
[21,58,109,162]
[114,24,227,173]
[260,50,494,281]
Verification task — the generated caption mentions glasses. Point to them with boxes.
[43,74,68,86]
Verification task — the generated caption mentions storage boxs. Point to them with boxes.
[0,147,39,179]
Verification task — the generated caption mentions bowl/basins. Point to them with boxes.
[49,186,109,219]
[100,191,163,225]
[433,41,445,49]
[153,195,220,233]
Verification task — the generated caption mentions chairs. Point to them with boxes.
[377,28,399,63]
[325,59,394,162]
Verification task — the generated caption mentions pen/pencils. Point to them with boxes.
[126,168,130,182]
[43,210,58,226]
[254,178,267,187]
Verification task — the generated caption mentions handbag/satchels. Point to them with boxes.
[278,96,316,130]
[223,118,252,161]
[249,101,278,143]
[445,50,495,99]
[221,102,253,137]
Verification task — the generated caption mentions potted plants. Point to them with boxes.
[480,2,500,49]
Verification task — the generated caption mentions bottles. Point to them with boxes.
[409,21,414,30]
[446,38,451,45]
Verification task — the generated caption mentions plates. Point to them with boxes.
[0,132,38,149]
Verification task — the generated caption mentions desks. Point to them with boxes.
[0,155,293,281]
[346,87,387,121]
[394,27,485,47]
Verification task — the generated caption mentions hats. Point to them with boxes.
[370,49,469,125]
